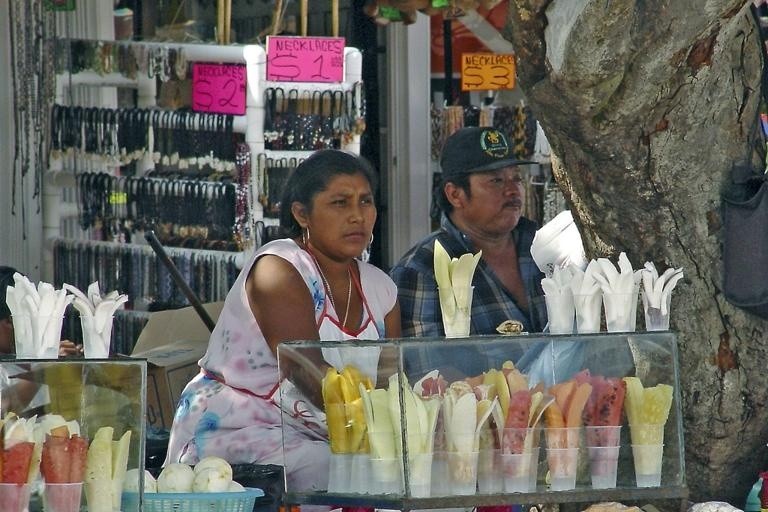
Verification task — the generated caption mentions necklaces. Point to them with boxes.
[308,250,353,329]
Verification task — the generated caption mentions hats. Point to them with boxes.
[440,126,540,175]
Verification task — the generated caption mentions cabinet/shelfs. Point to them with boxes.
[40,36,364,306]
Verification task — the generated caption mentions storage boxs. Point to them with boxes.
[117,301,226,433]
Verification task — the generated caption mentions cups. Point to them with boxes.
[542,293,673,336]
[1,480,123,511]
[584,424,623,489]
[628,423,665,488]
[543,427,580,491]
[10,314,116,360]
[436,286,474,339]
[325,426,541,495]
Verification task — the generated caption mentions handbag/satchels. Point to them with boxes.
[718,112,768,307]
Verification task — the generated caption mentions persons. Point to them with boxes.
[388,125,589,387]
[0,266,84,419]
[161,149,402,512]
